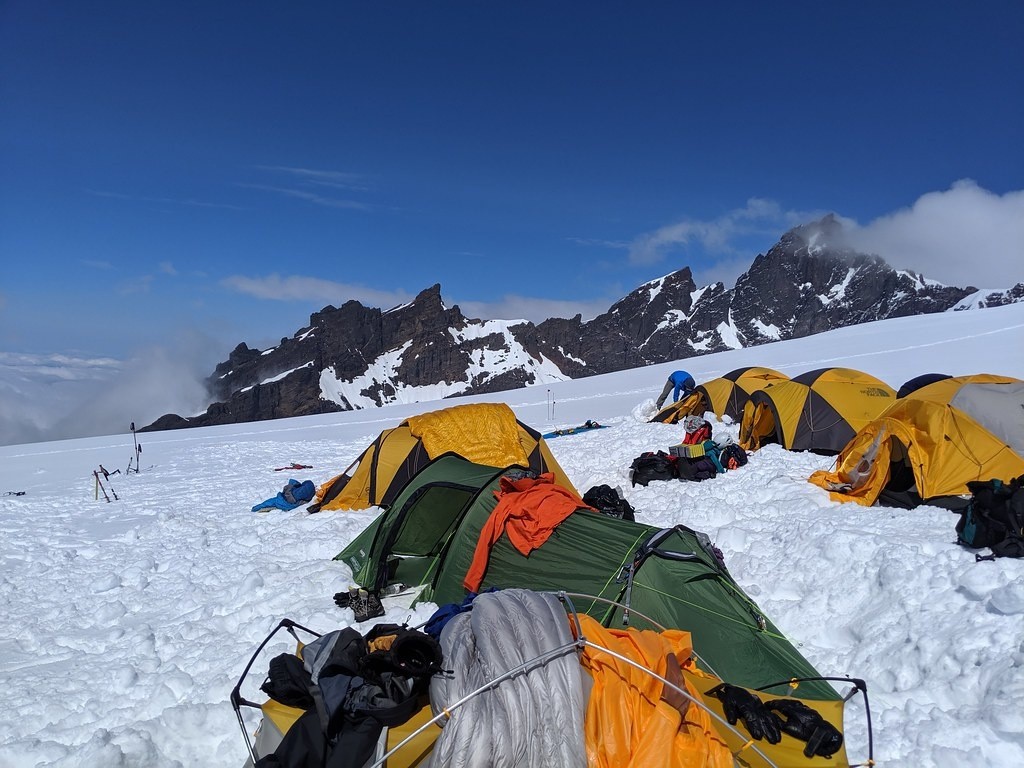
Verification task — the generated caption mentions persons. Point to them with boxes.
[657,370,695,410]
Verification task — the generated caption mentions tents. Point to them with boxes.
[649,367,790,424]
[231,590,874,767]
[806,374,1024,508]
[331,451,842,701]
[318,403,582,499]
[740,368,897,456]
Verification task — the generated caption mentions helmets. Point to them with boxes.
[713,431,733,450]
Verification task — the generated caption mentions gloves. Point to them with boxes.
[334,591,349,607]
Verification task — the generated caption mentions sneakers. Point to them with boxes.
[345,587,385,622]
